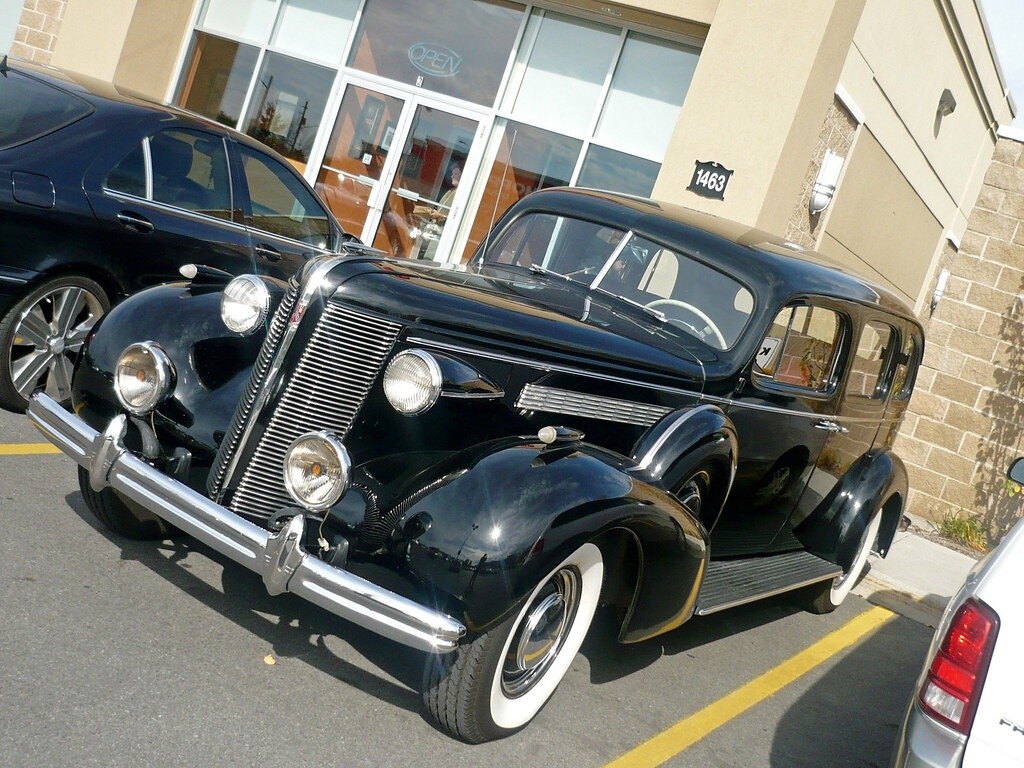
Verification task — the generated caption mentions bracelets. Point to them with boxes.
[442,215,446,220]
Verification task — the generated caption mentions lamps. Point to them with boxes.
[931,267,949,308]
[939,89,957,116]
[809,148,846,215]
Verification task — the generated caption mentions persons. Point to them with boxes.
[417,159,465,261]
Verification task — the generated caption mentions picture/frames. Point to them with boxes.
[348,94,386,161]
[398,116,433,179]
[376,120,396,156]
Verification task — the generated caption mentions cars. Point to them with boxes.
[1,52,377,416]
[893,452,1022,768]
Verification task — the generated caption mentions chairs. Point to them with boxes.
[150,133,206,210]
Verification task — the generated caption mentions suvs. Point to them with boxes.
[24,185,924,746]
